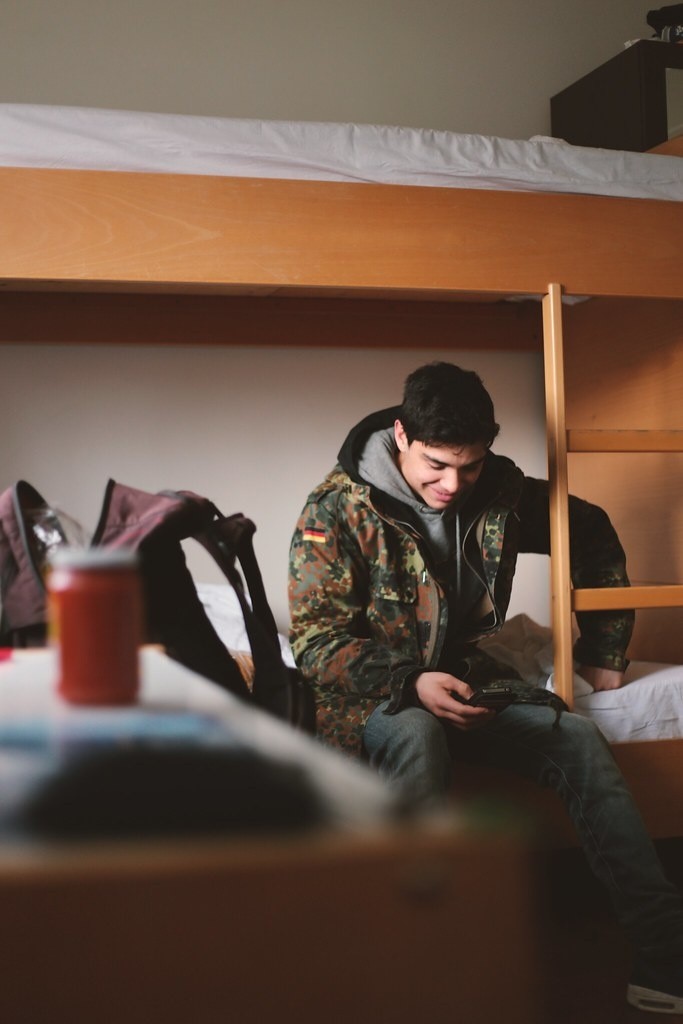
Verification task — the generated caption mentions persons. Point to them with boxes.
[288,359,683,1016]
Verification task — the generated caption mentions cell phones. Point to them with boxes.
[466,686,514,713]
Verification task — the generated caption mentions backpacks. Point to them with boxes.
[0,476,316,759]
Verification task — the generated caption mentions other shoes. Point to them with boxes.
[626,955,683,1015]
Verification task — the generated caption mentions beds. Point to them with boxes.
[0,101,683,847]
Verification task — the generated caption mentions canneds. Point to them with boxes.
[46,546,140,705]
